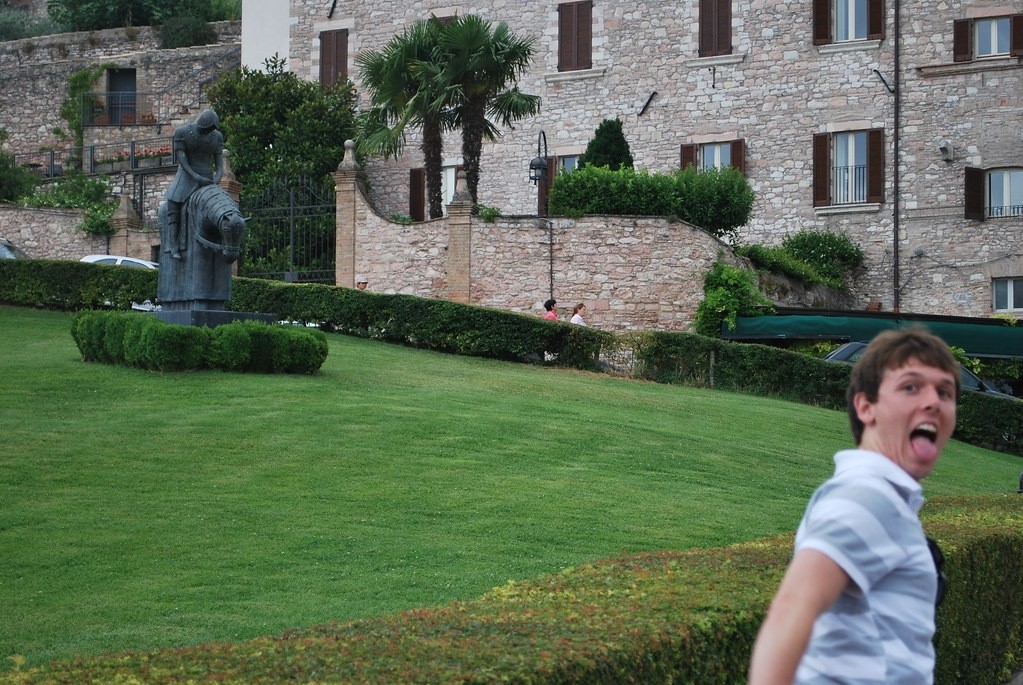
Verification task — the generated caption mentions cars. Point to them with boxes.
[80,254,162,312]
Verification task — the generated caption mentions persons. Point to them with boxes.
[750,329,962,684]
[543,299,556,319]
[357,278,368,290]
[570,304,587,325]
[166,109,223,258]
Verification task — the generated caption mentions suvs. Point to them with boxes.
[0,237,31,259]
[825,340,1022,401]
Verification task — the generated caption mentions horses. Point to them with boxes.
[157,184,252,311]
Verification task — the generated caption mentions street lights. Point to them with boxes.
[528,130,554,300]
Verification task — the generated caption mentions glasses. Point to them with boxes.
[926,534,949,609]
